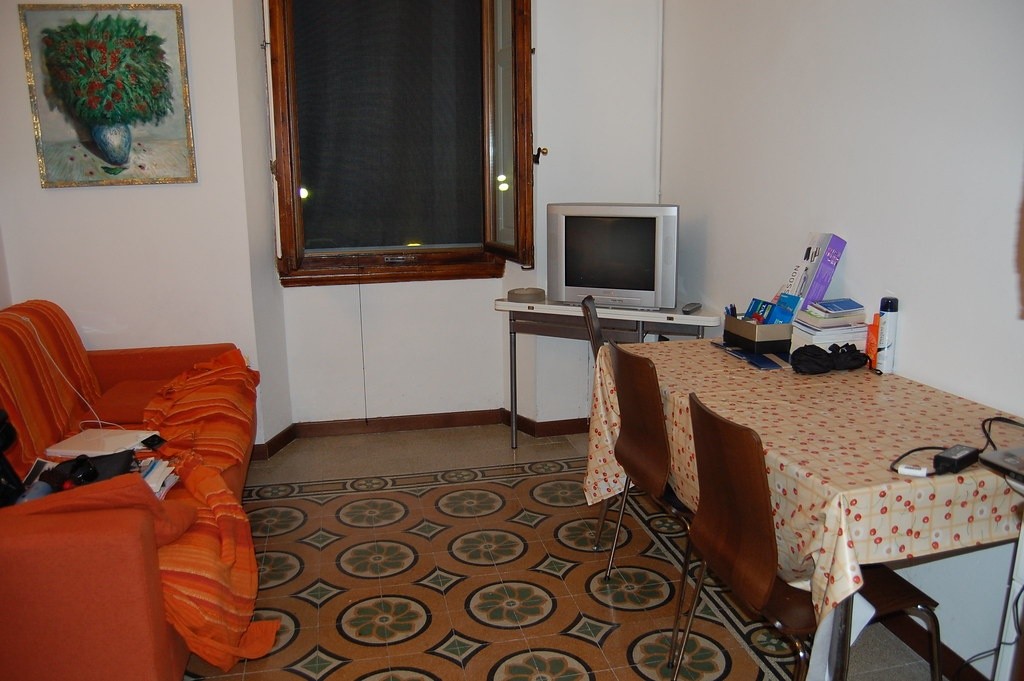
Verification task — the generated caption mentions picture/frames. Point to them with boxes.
[18,4,198,188]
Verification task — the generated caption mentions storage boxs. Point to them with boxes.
[745,232,847,324]
[723,313,793,354]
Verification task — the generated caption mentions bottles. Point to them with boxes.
[865,314,880,369]
[875,297,899,374]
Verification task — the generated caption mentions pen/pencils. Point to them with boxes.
[704,339,732,351]
[724,303,737,318]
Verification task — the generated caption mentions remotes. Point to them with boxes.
[682,303,702,315]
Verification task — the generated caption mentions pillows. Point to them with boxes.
[0,472,198,547]
[80,378,172,424]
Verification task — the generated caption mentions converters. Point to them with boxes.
[933,445,980,473]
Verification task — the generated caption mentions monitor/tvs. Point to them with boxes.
[547,203,679,312]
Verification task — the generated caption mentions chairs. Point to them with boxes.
[591,337,940,681]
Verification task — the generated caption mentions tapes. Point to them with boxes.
[507,286,546,303]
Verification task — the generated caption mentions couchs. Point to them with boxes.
[0,299,260,681]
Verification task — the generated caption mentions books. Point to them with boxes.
[789,297,868,354]
[22,427,180,499]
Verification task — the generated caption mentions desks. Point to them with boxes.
[583,337,1024,681]
[495,298,721,449]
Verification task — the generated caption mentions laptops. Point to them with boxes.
[46,429,160,459]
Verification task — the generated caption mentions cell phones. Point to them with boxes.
[141,434,167,451]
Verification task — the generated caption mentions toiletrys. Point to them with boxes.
[865,295,899,374]
